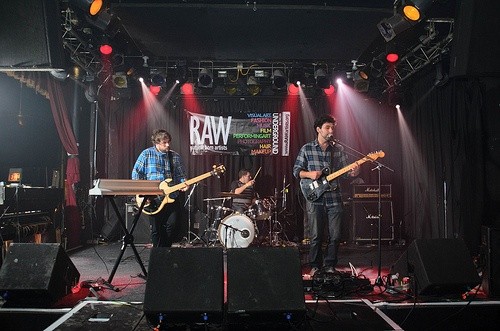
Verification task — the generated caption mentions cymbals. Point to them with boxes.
[217,191,240,197]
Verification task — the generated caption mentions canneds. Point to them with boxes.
[402,277,411,293]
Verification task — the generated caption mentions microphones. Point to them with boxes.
[327,133,339,142]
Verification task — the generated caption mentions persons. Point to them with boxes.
[131,129,189,249]
[228,169,257,215]
[292,114,361,279]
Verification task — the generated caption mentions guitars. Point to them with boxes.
[299,149,386,204]
[135,162,227,216]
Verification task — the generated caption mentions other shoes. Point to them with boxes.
[310,267,320,277]
[326,264,336,273]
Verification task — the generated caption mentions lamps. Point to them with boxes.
[80,0,115,31]
[290,66,306,86]
[386,43,399,62]
[403,3,425,24]
[313,64,331,89]
[100,35,112,54]
[112,69,127,89]
[112,53,125,66]
[349,57,383,92]
[377,15,409,42]
[197,62,214,88]
[271,62,286,90]
[255,70,264,77]
[217,70,228,78]
[173,61,188,84]
[137,63,150,85]
[150,71,166,86]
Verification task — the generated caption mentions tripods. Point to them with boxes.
[334,138,414,299]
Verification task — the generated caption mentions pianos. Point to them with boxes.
[88,178,164,292]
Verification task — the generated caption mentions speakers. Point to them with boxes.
[125,203,153,248]
[392,237,481,300]
[227,247,306,320]
[351,200,394,245]
[0,241,80,305]
[145,244,224,322]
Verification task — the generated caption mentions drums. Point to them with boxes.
[204,198,231,221]
[250,199,271,221]
[216,213,259,249]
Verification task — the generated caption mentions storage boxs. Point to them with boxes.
[352,200,395,243]
